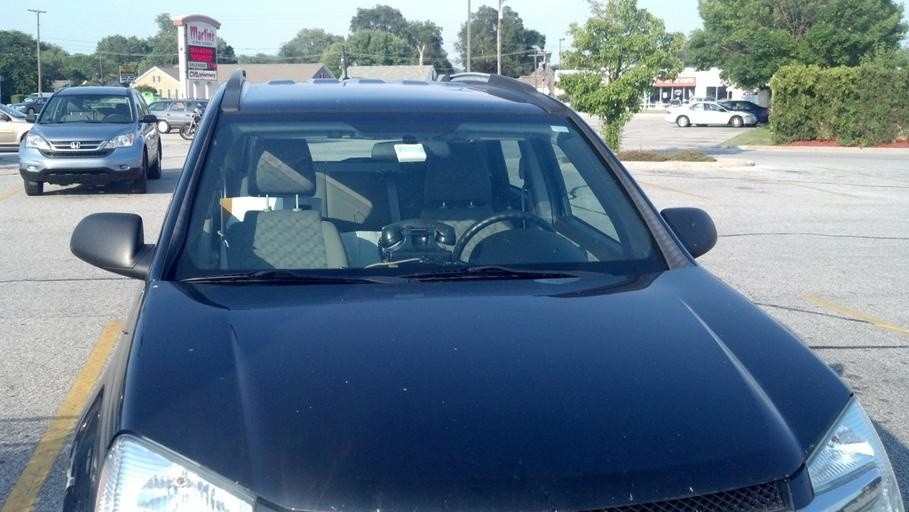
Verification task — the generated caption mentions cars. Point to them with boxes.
[715,100,769,127]
[1,86,209,196]
[70,69,907,511]
[665,100,758,128]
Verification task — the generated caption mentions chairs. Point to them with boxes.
[61,99,130,123]
